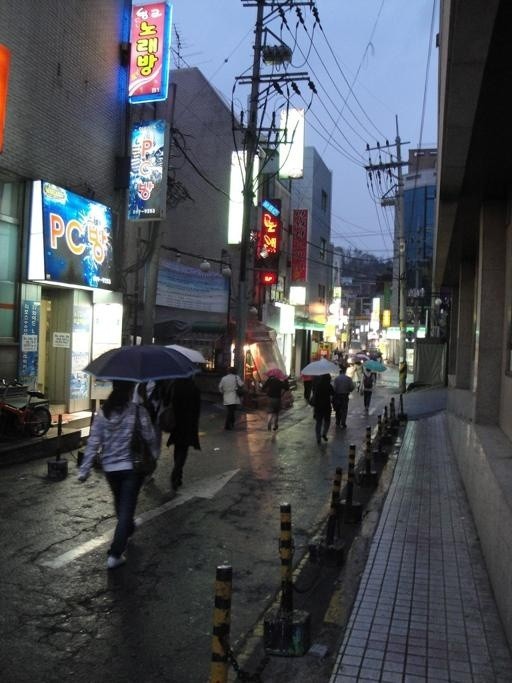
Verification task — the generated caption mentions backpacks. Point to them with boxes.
[364,372,373,388]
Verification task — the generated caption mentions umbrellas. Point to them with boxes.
[353,349,387,372]
[83,345,201,382]
[165,343,208,364]
[301,359,342,376]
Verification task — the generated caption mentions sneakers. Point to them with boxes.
[133,518,144,527]
[107,554,126,568]
[170,469,183,491]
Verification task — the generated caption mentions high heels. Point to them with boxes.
[317,434,328,443]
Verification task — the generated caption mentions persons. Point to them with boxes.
[78,380,161,569]
[335,368,354,428]
[167,377,201,484]
[313,374,334,444]
[263,376,287,430]
[340,354,382,411]
[217,367,244,430]
[132,380,163,488]
[301,375,314,401]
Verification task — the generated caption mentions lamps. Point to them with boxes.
[196,254,233,277]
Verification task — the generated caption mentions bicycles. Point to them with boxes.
[0,376,51,437]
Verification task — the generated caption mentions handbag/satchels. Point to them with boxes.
[237,386,246,396]
[155,401,175,432]
[309,391,319,406]
[333,399,342,411]
[130,430,158,476]
